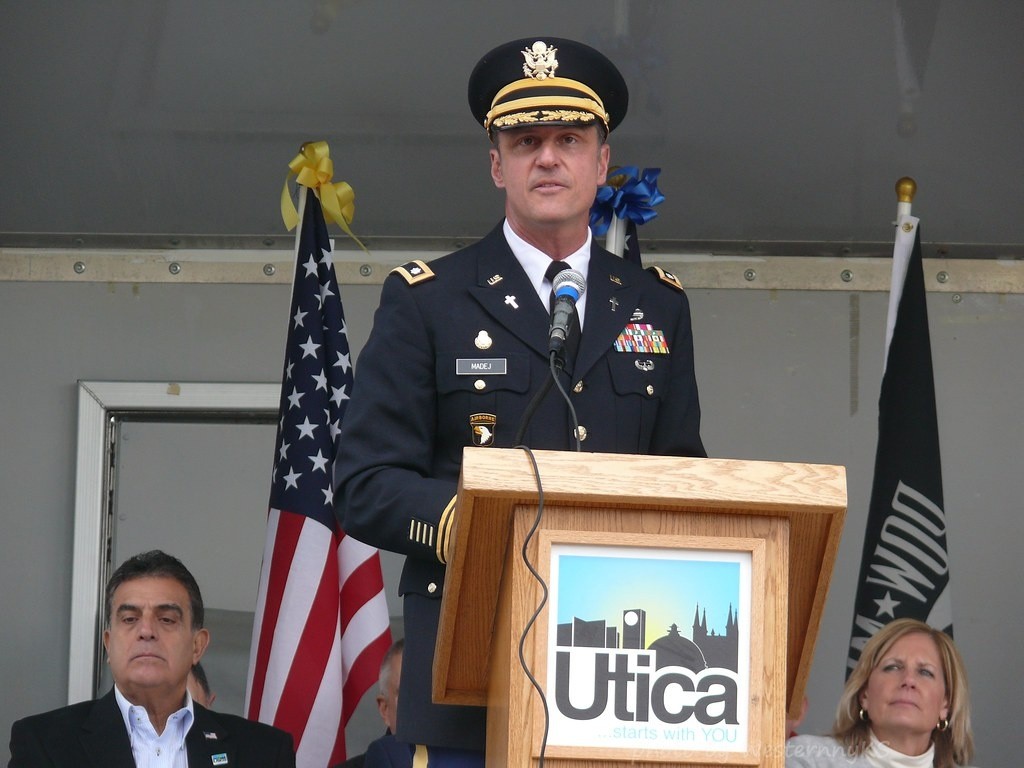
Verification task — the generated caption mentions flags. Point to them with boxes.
[844,214,954,686]
[242,174,394,767]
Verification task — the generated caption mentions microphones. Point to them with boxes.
[550,269,587,355]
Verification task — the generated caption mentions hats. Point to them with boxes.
[466,35,629,142]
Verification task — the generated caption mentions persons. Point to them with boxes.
[786,617,975,768]
[334,638,485,768]
[6,548,297,768]
[329,36,710,768]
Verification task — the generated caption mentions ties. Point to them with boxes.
[545,260,581,366]
